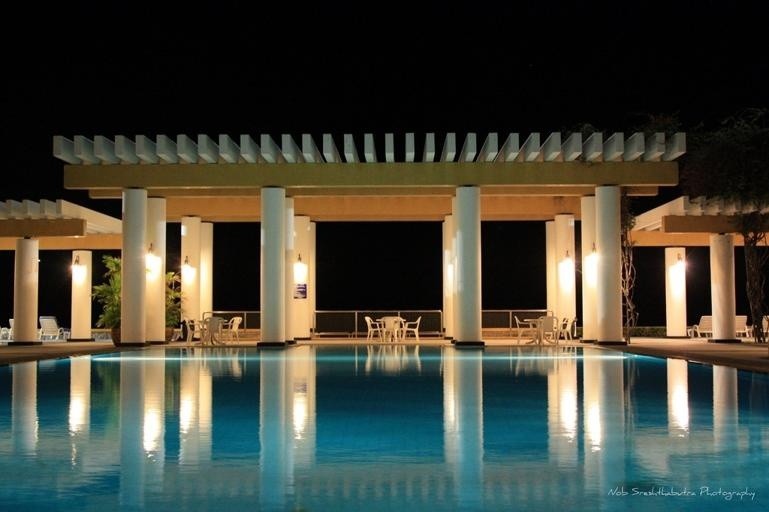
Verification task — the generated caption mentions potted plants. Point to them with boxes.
[163,271,186,345]
[90,252,122,348]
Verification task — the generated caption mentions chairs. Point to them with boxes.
[38,315,66,342]
[0,319,14,342]
[182,314,245,346]
[364,313,424,342]
[512,315,579,347]
[686,314,768,339]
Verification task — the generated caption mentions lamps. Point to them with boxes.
[73,255,81,265]
[675,252,684,261]
[297,253,301,263]
[590,242,599,253]
[184,255,190,265]
[564,250,571,259]
[147,243,156,254]
